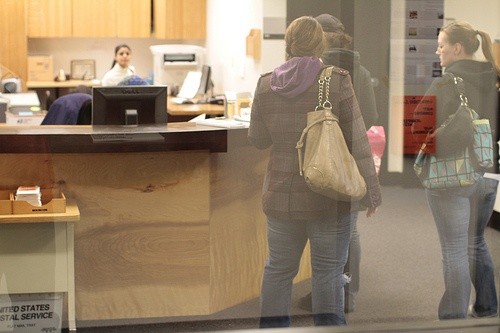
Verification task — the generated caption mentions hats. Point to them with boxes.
[117,74,151,85]
[315,12,346,32]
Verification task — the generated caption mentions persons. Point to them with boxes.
[248,17,382,330]
[299,13,379,315]
[102,45,136,86]
[412,22,500,321]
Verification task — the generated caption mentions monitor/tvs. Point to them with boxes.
[91,87,168,126]
[199,65,214,97]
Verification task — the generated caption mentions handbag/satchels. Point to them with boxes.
[427,74,475,160]
[412,73,494,188]
[296,66,367,202]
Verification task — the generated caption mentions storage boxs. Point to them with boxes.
[0,187,66,214]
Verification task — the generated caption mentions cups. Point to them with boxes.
[223,99,241,120]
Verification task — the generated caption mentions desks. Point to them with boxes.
[0,199,81,333]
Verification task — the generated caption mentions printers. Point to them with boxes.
[151,45,208,96]
